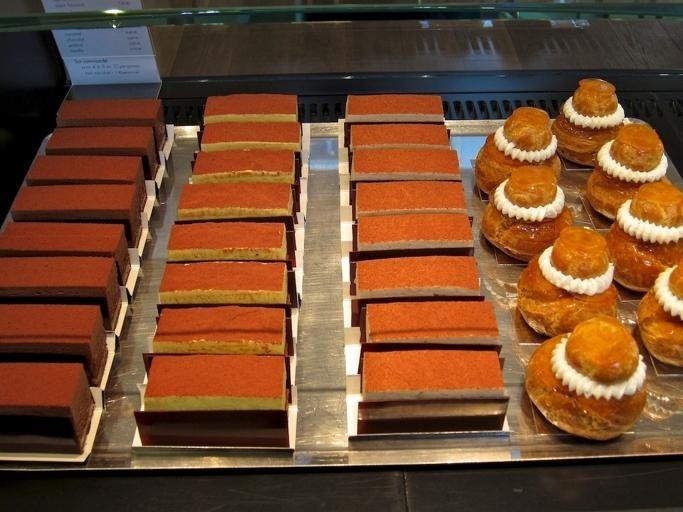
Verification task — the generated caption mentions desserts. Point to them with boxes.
[343,95,509,433]
[0,92,168,454]
[144,94,302,447]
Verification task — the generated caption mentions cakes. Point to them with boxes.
[518,227,620,336]
[483,164,573,262]
[586,124,672,221]
[526,314,647,440]
[603,182,683,291]
[634,264,682,369]
[551,79,625,166]
[474,107,561,195]
